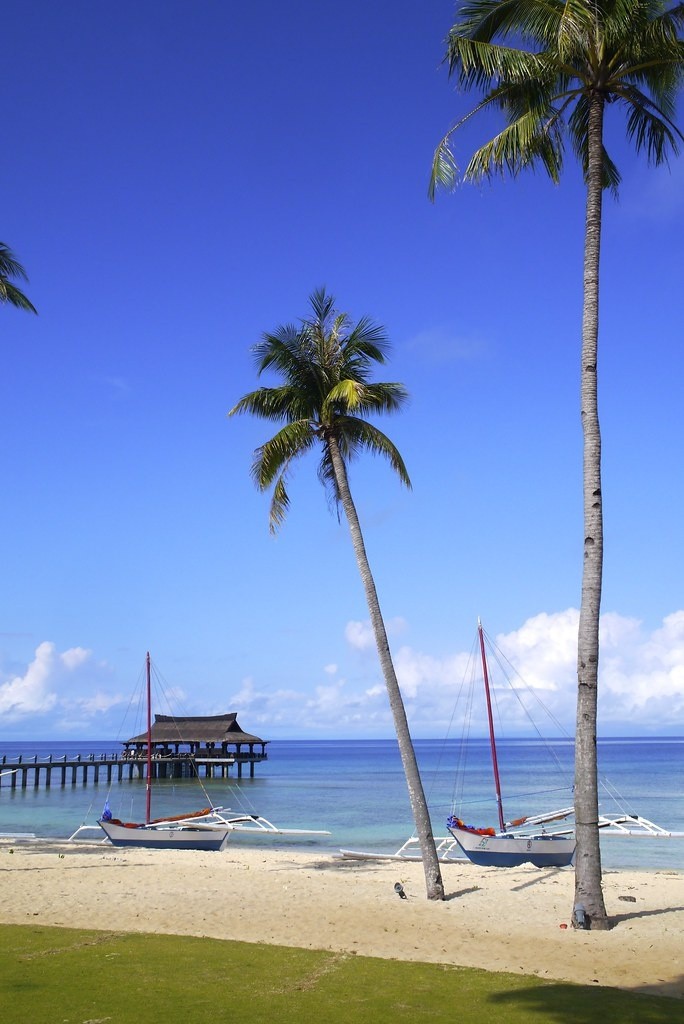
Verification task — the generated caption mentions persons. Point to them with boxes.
[130,749,135,758]
[122,750,128,756]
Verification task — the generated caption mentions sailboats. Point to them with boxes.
[95,649,230,850]
[445,617,579,867]
